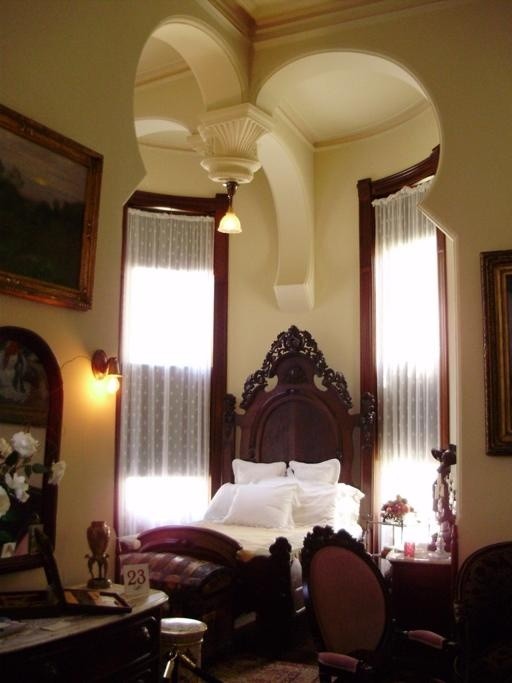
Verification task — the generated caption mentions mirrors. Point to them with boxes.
[0,325,63,576]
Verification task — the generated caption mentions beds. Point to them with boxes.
[118,323,379,666]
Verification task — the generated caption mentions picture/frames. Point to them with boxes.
[480,251,512,456]
[1,103,104,310]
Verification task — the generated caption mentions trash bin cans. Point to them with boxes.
[144,617,207,683]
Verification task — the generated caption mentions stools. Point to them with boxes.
[160,617,222,683]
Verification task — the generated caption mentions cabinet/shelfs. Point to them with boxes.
[0,577,170,681]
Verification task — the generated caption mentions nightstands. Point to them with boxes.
[386,545,454,631]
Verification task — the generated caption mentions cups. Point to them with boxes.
[404,540,416,558]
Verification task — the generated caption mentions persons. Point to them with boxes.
[23,354,47,402]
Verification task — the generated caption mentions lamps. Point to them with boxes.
[90,350,122,393]
[218,182,241,234]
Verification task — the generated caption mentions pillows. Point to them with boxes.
[224,482,300,528]
[290,459,340,485]
[205,485,231,523]
[332,484,364,540]
[295,481,332,528]
[224,458,284,485]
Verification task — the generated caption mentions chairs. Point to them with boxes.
[300,525,459,682]
[457,539,512,683]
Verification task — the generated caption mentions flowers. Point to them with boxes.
[0,431,65,515]
[380,494,414,522]
